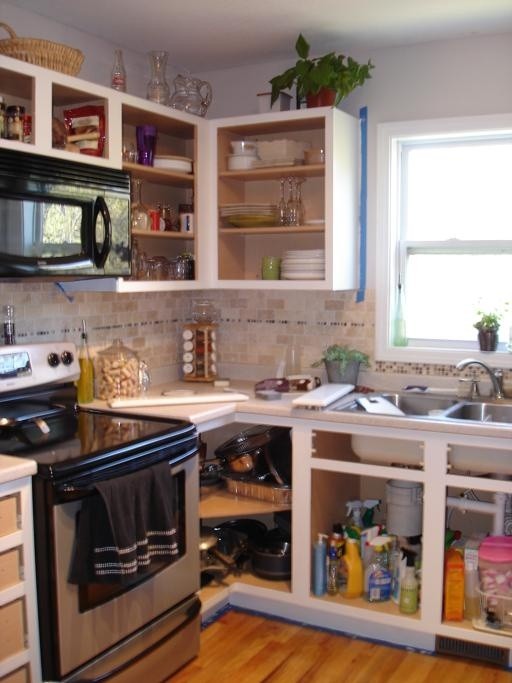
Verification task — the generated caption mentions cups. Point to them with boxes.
[136,122,158,168]
[262,256,281,279]
[277,177,305,226]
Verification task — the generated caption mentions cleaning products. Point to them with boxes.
[326,547,340,595]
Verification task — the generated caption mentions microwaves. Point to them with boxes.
[0,147,132,279]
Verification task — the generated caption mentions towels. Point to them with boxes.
[66,458,182,586]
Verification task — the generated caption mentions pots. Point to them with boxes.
[214,422,291,490]
[251,527,291,580]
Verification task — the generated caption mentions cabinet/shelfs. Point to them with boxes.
[194,412,512,647]
[0,54,357,292]
[0,454,42,683]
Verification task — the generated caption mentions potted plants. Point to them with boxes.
[312,340,370,386]
[473,301,510,351]
[267,32,375,108]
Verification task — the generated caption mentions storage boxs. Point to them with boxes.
[472,585,512,636]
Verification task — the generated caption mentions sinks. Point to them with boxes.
[442,396,512,427]
[332,389,461,419]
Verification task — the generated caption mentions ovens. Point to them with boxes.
[0,343,202,683]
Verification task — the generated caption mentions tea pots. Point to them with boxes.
[170,75,212,117]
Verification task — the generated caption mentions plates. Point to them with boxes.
[222,474,290,505]
[251,158,304,168]
[154,155,193,173]
[221,203,280,227]
[280,249,325,281]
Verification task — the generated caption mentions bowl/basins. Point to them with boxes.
[256,138,310,161]
[230,141,259,154]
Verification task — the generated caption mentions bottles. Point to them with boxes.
[95,339,141,401]
[1,305,16,345]
[112,48,127,91]
[129,179,150,231]
[0,102,7,140]
[131,238,141,281]
[8,105,25,142]
[75,331,93,405]
[24,115,33,144]
[466,563,482,620]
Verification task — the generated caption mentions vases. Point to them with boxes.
[146,49,170,105]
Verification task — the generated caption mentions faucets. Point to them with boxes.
[455,357,508,399]
